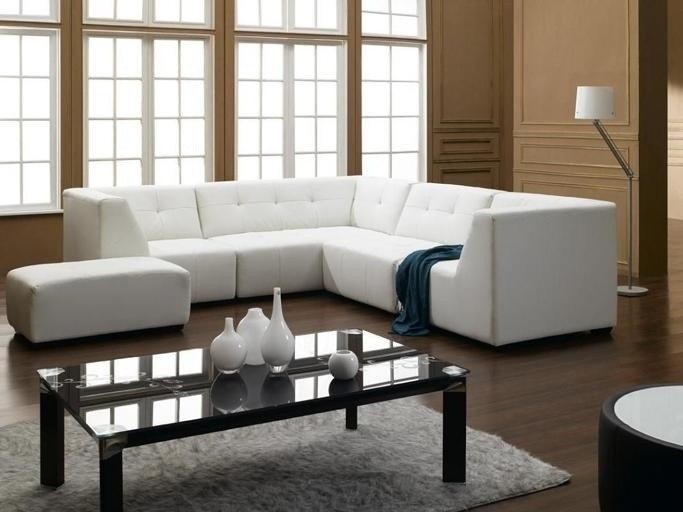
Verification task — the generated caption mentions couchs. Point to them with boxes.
[60,175,621,347]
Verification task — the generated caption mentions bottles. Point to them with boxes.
[209,285,294,377]
[326,350,360,381]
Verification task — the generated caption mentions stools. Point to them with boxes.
[5,255,193,360]
[597,381,683,512]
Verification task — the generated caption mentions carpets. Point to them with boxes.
[1,397,574,511]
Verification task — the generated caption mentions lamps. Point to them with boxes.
[575,84,650,298]
